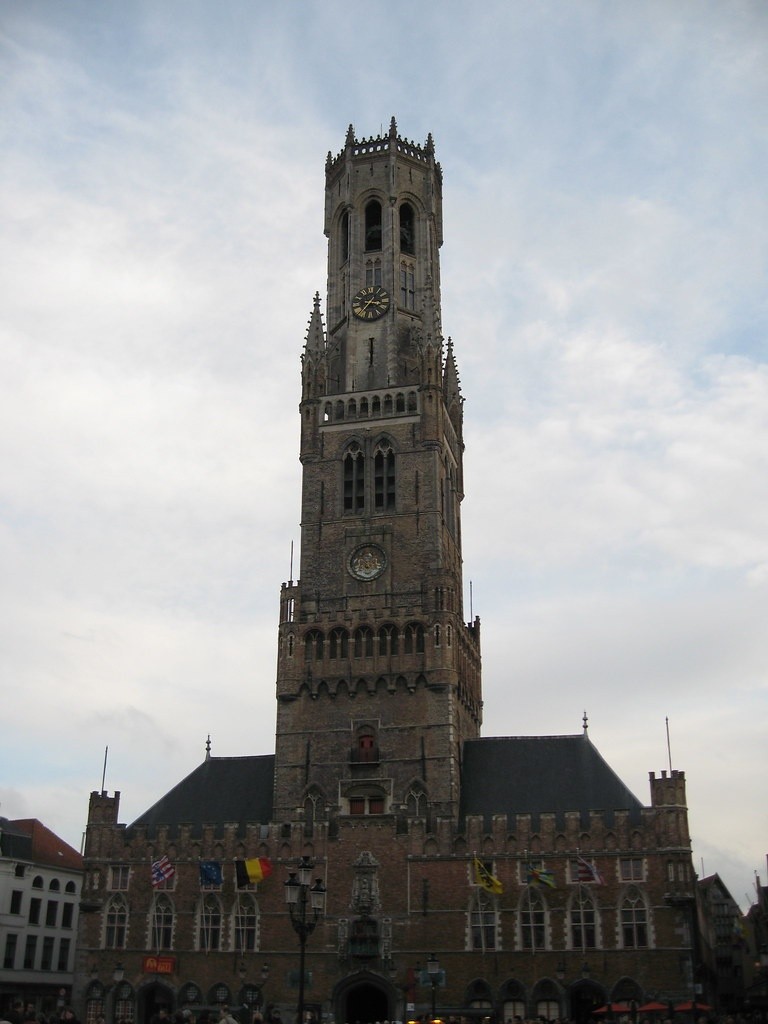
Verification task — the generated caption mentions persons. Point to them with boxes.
[0,1005,768,1024]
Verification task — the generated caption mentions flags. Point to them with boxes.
[475,858,502,894]
[150,856,176,888]
[235,856,272,889]
[578,858,608,886]
[528,864,556,891]
[199,861,224,886]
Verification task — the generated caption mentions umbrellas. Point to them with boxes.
[591,1001,713,1018]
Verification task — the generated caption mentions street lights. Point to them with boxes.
[426,952,441,1020]
[237,959,271,1024]
[283,855,328,1024]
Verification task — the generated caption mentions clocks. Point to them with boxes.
[351,286,390,321]
